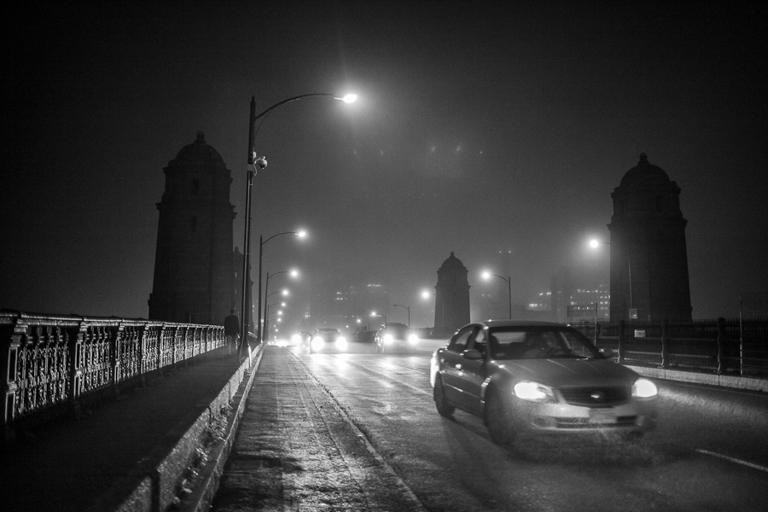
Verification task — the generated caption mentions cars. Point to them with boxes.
[292,329,348,353]
[429,318,659,451]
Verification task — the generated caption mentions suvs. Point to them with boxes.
[373,321,420,354]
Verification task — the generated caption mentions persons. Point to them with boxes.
[482,336,510,359]
[521,332,552,360]
[224,309,239,356]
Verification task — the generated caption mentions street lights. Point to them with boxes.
[237,86,360,357]
[481,271,511,320]
[336,304,411,338]
[256,228,307,344]
[590,238,634,317]
[263,268,296,341]
